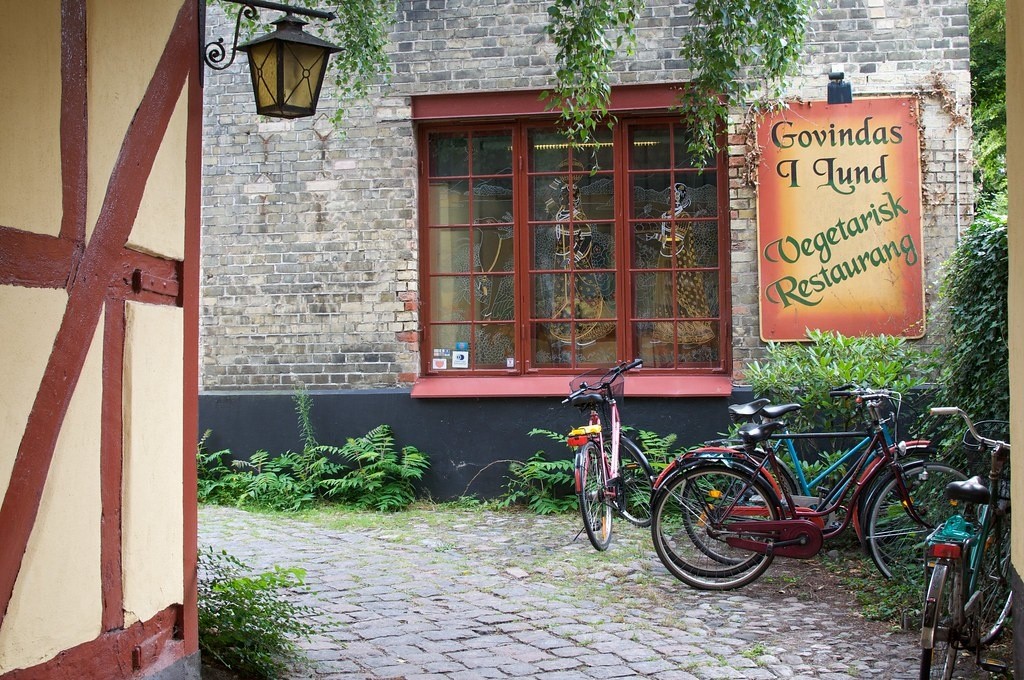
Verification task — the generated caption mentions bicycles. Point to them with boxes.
[920,404,1015,680]
[649,383,968,591]
[560,359,656,552]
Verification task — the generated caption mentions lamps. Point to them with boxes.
[827,72,853,105]
[197,0,346,120]
